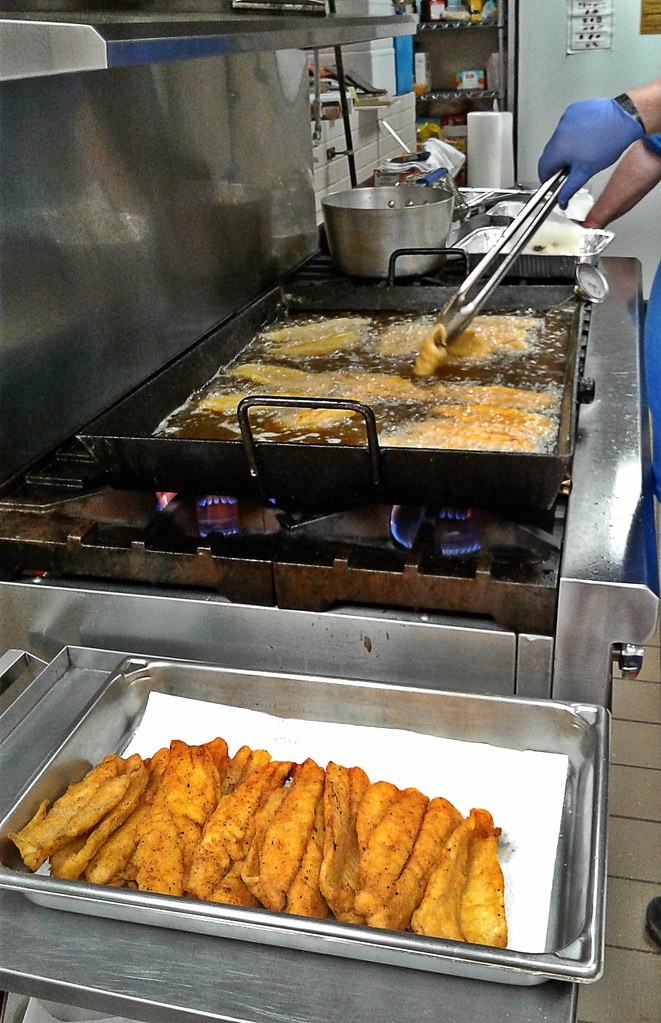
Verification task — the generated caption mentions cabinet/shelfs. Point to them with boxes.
[395,0,507,191]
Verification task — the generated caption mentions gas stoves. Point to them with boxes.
[0,281,656,708]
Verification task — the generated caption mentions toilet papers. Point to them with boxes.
[465,110,521,192]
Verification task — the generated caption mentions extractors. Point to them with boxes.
[0,1,415,81]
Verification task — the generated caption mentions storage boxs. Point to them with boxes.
[0,653,612,988]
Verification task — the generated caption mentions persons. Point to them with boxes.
[538,81,660,505]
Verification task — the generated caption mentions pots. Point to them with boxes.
[321,168,453,281]
[73,245,589,510]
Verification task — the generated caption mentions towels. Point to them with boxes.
[419,134,468,182]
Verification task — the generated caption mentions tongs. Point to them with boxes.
[429,163,572,343]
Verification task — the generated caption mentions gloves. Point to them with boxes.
[539,98,647,211]
